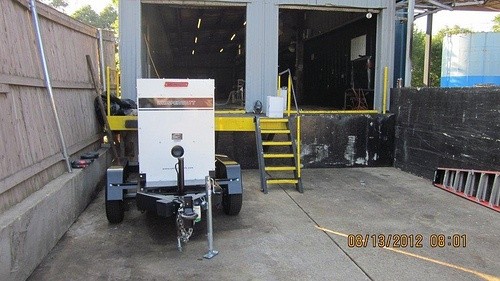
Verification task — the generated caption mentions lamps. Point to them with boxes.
[452,1,484,7]
[288,42,297,52]
[366,12,372,18]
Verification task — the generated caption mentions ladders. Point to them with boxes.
[432,166,500,213]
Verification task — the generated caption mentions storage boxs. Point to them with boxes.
[266,96,283,118]
